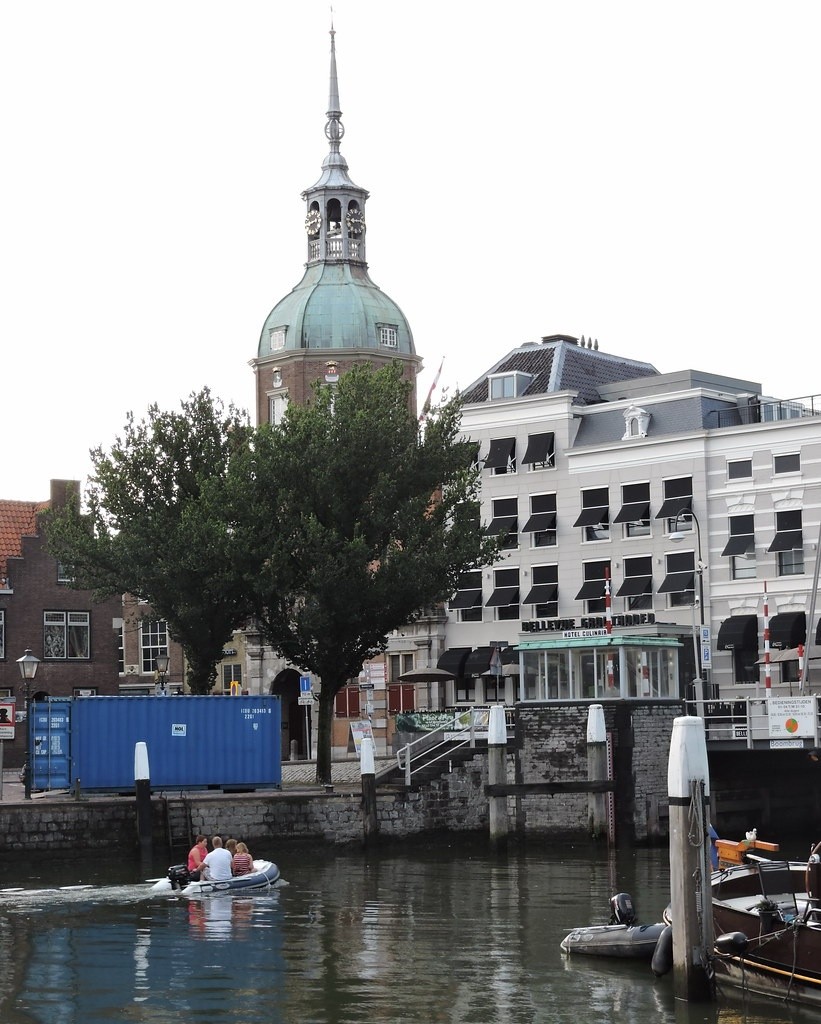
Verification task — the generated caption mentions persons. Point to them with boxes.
[186,834,258,881]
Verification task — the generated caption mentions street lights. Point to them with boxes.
[152,649,171,699]
[668,508,712,738]
[15,648,41,800]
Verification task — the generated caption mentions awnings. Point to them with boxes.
[436,646,500,677]
[770,612,805,650]
[716,614,757,651]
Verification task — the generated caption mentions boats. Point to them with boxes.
[151,858,280,898]
[657,827,821,1016]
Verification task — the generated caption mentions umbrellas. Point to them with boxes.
[397,668,456,711]
